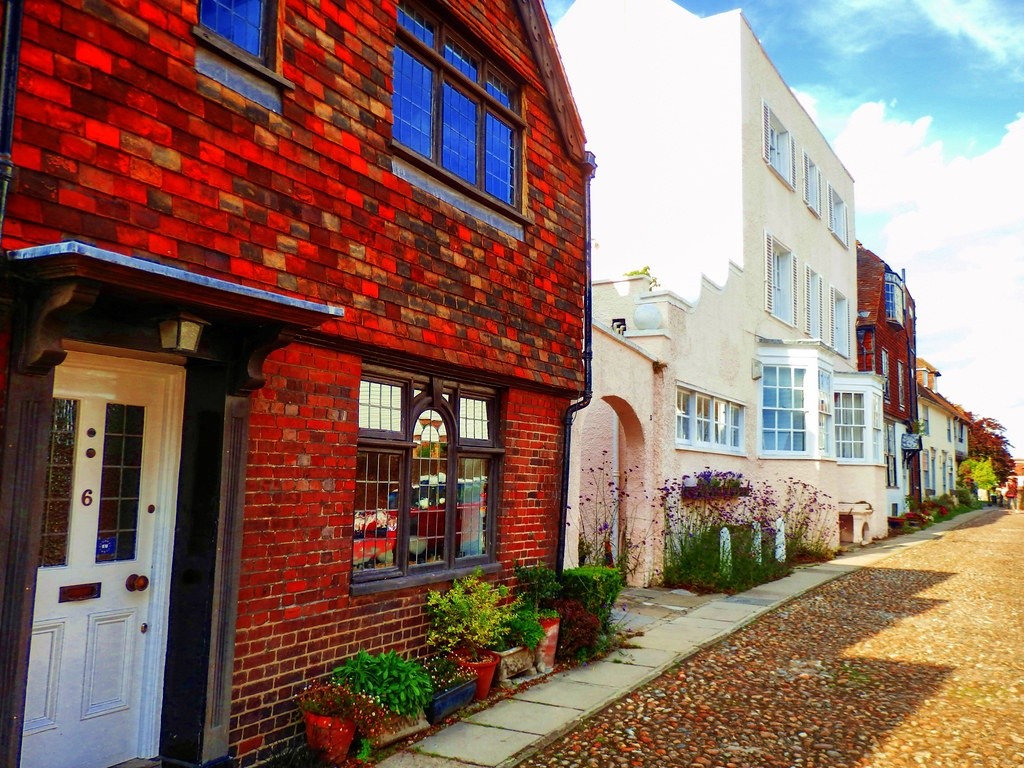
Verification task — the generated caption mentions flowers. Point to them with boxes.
[292,672,395,752]
[411,650,478,693]
[905,513,927,528]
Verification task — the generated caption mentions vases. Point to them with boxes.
[888,516,906,529]
[302,710,359,765]
[425,675,480,726]
[907,519,921,529]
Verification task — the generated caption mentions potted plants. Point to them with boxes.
[332,645,432,749]
[492,593,546,685]
[423,563,529,701]
[511,558,562,672]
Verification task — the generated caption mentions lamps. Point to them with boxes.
[154,301,214,354]
